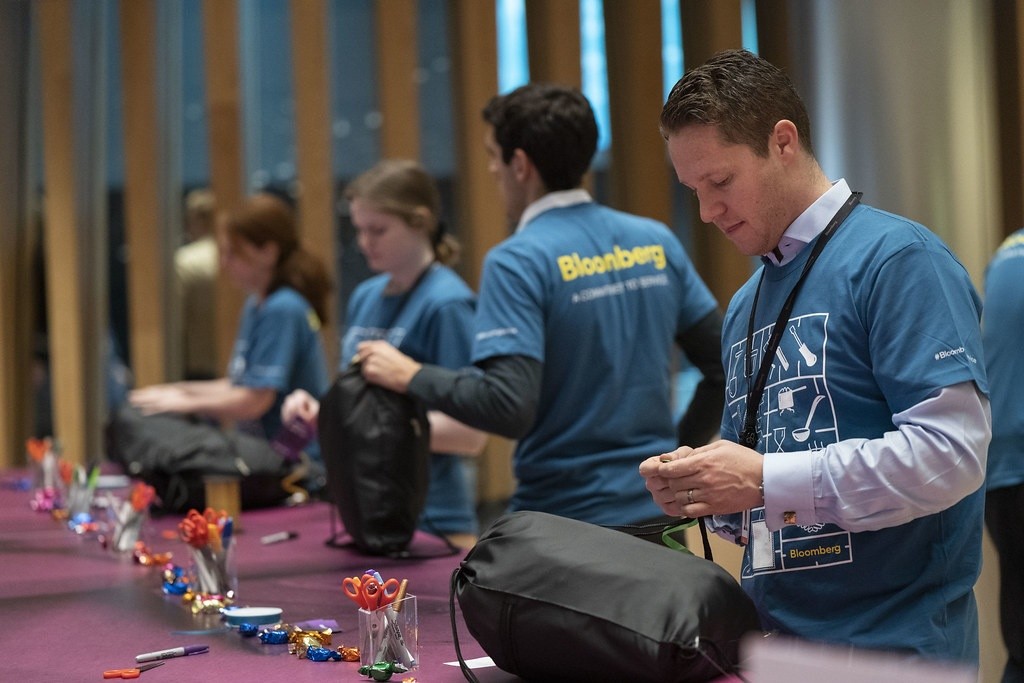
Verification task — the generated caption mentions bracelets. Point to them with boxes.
[760,480,764,499]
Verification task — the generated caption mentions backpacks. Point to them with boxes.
[316,372,433,555]
[103,409,289,516]
[449,510,764,681]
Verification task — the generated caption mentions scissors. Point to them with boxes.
[178,507,229,596]
[103,660,164,680]
[342,574,400,666]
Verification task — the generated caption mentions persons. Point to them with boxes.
[281,158,489,558]
[352,85,726,554]
[168,184,331,424]
[985,227,1023,683]
[640,48,992,683]
[128,190,331,498]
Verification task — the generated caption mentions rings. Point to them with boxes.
[688,490,694,503]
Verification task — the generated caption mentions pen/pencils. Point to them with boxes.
[365,570,414,668]
[218,517,234,569]
[134,644,209,663]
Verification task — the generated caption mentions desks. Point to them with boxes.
[0,463,752,683]
